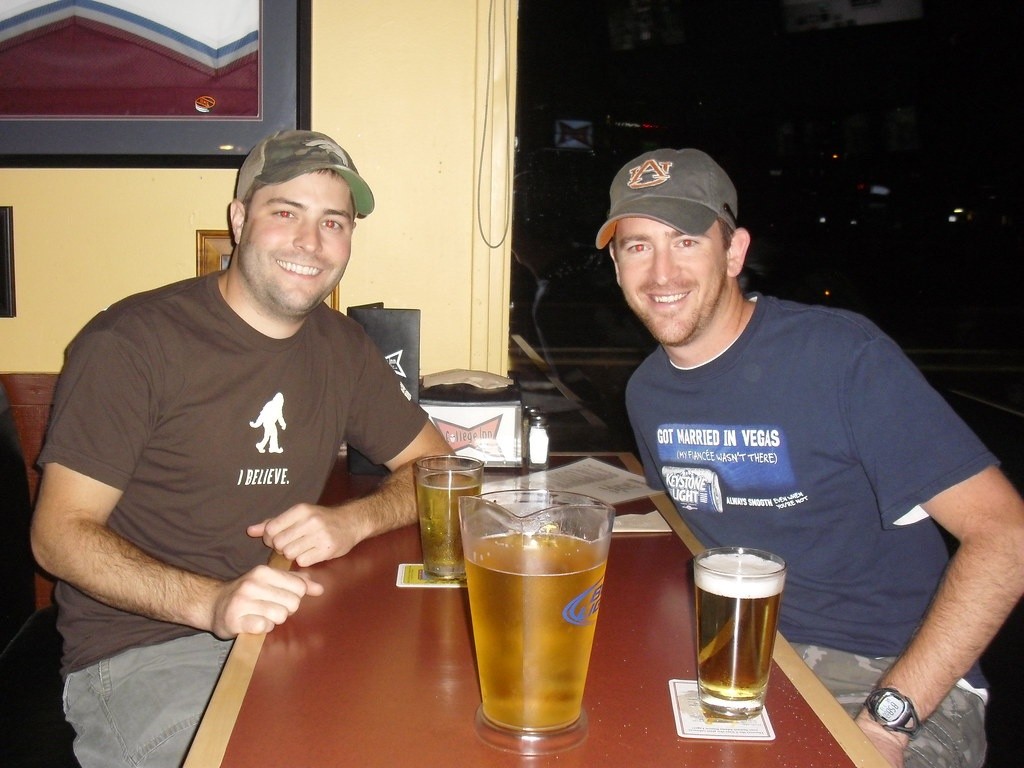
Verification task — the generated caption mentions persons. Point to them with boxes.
[596,148,1024,767]
[31,130,455,768]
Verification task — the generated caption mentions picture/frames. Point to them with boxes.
[196,230,340,311]
[0,0,300,158]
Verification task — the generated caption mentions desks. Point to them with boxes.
[180,450,890,768]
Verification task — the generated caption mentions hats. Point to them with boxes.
[237,130,375,218]
[596,147,738,249]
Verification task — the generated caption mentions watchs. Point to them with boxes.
[864,689,924,739]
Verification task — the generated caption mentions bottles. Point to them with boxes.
[519,405,549,471]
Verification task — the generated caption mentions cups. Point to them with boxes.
[692,547,787,723]
[414,455,485,583]
[458,489,616,758]
